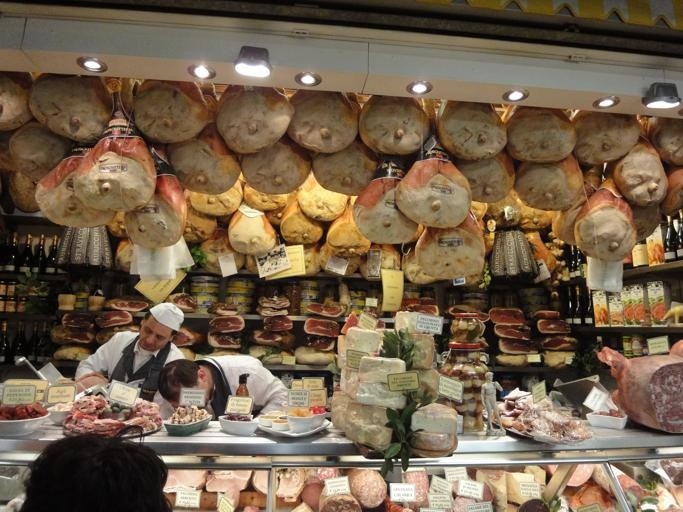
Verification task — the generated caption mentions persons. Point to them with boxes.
[20,433,171,510]
[73,302,185,420]
[157,354,290,418]
[481,369,506,430]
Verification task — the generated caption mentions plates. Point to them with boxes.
[164,415,211,435]
[218,415,257,436]
[582,410,627,430]
[253,410,331,440]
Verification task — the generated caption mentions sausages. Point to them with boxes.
[548,463,646,504]
[301,468,412,512]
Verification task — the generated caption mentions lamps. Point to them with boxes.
[233,46,270,80]
[642,82,680,110]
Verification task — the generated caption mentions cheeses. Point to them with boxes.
[476,465,546,511]
[332,309,459,458]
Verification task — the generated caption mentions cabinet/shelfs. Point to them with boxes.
[595,263,683,340]
[114,310,578,377]
[2,207,67,365]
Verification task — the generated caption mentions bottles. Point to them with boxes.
[236,373,249,398]
[0,230,65,275]
[563,207,682,280]
[562,284,596,330]
[620,334,643,358]
[0,320,57,366]
[0,279,50,313]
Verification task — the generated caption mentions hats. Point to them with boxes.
[149,302,184,332]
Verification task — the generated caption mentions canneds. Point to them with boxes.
[439,343,489,432]
[446,288,518,310]
[622,332,644,358]
[451,313,486,343]
[0,279,27,313]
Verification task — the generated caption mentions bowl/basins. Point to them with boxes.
[0,411,52,442]
[47,406,69,425]
